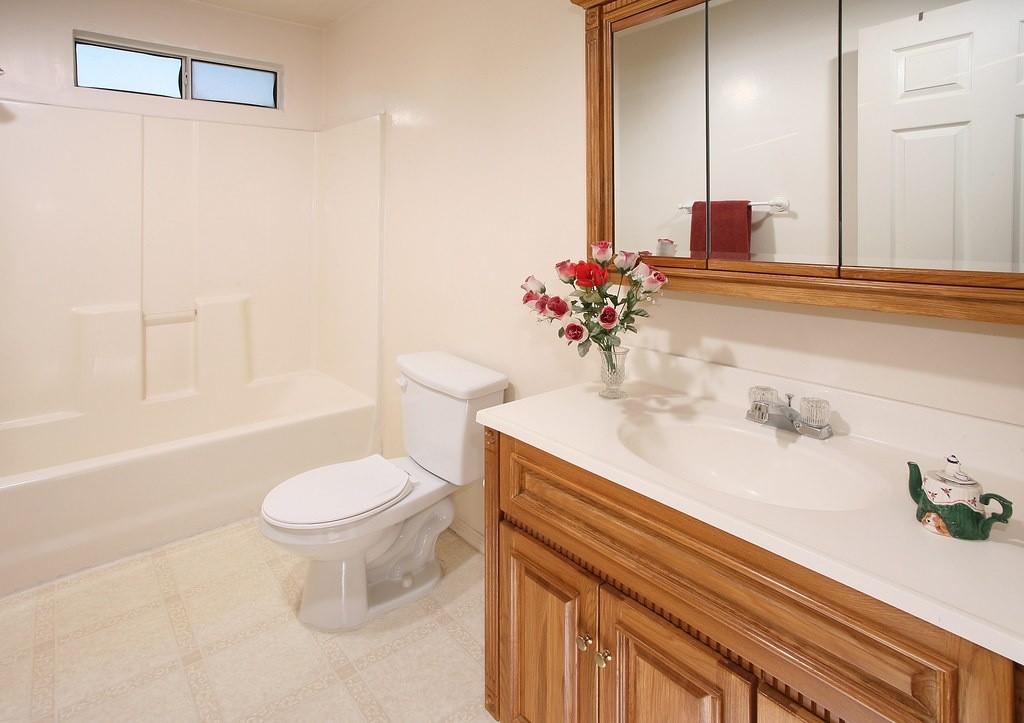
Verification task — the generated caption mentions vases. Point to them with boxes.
[596,344,630,399]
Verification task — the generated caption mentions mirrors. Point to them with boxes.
[610,0,1024,271]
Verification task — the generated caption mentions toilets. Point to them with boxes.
[257,345,511,639]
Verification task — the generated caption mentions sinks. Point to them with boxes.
[615,412,898,514]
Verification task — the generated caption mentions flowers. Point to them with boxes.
[522,237,668,373]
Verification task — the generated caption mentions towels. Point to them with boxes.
[689,199,755,263]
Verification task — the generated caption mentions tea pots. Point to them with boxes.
[906,454,1013,541]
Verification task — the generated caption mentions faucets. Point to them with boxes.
[744,385,834,441]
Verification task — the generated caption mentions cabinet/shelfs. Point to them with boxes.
[573,0,1024,326]
[491,517,837,723]
